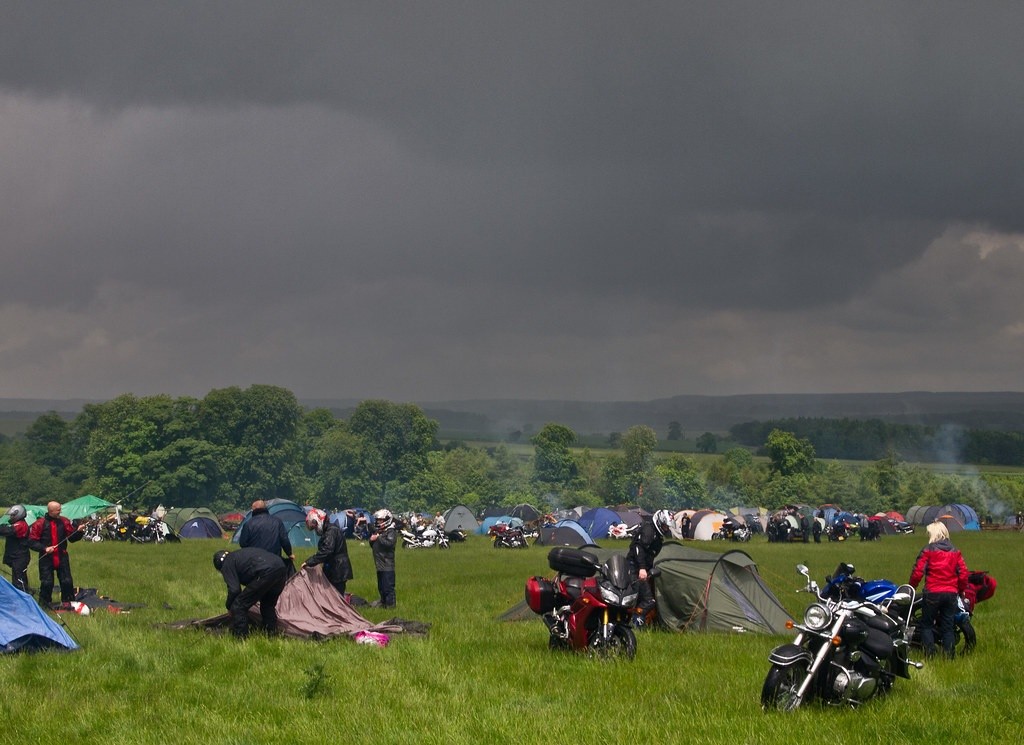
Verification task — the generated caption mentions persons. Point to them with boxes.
[369,508,397,608]
[29,501,86,609]
[765,510,842,542]
[985,510,992,524]
[345,510,445,539]
[303,508,353,598]
[0,506,30,592]
[909,522,968,662]
[239,500,295,559]
[1016,511,1021,524]
[626,509,676,630]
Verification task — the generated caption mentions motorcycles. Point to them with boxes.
[889,519,915,534]
[448,525,467,542]
[825,517,853,543]
[488,521,530,550]
[72,507,166,546]
[760,566,925,714]
[400,520,451,551]
[820,561,976,658]
[605,521,641,540]
[712,515,762,543]
[524,547,661,663]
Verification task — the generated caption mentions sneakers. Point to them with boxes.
[632,615,645,631]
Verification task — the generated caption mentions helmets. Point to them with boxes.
[306,508,327,537]
[213,550,231,571]
[4,504,27,525]
[651,509,675,538]
[373,509,393,530]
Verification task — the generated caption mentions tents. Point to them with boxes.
[497,541,801,636]
[443,504,655,546]
[231,498,374,548]
[671,506,771,540]
[214,549,286,638]
[157,565,431,640]
[0,576,79,654]
[769,505,842,534]
[0,494,226,540]
[833,504,980,530]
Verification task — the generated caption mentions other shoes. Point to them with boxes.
[372,601,395,610]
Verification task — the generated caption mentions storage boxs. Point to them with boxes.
[548,547,600,576]
[524,576,556,614]
